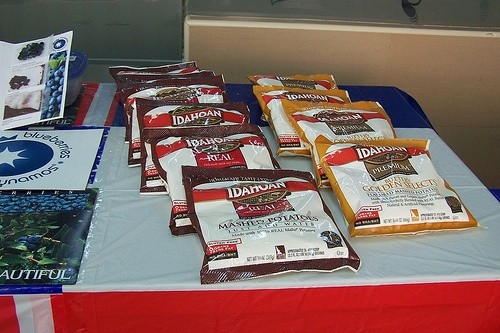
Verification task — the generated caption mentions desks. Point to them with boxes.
[0,81,499,332]
